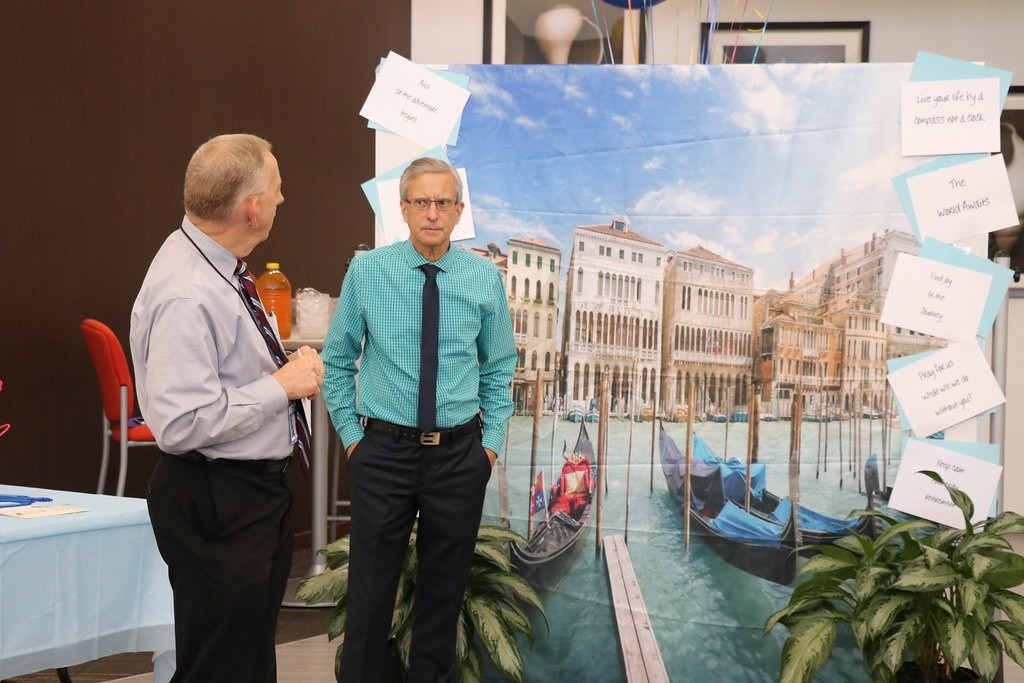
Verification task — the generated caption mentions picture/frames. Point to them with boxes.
[482,0,649,66]
[701,20,871,65]
[987,85,1024,299]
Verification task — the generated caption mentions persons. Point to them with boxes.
[320,157,518,683]
[130,133,326,683]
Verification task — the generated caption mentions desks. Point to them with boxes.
[0,482,179,683]
[280,298,365,608]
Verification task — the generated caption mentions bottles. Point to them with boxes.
[255,263,292,339]
[244,262,257,283]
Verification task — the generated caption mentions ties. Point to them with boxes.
[418,264,439,432]
[234,260,314,479]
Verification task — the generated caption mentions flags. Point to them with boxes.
[529,472,546,516]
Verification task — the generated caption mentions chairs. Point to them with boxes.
[80,318,157,500]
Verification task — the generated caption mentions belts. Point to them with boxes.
[366,414,479,446]
[161,452,290,477]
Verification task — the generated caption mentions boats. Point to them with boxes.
[569,409,600,424]
[658,417,792,547]
[510,416,596,564]
[691,429,880,544]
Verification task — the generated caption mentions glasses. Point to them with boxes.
[405,191,457,211]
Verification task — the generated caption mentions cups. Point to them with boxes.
[296,293,330,339]
